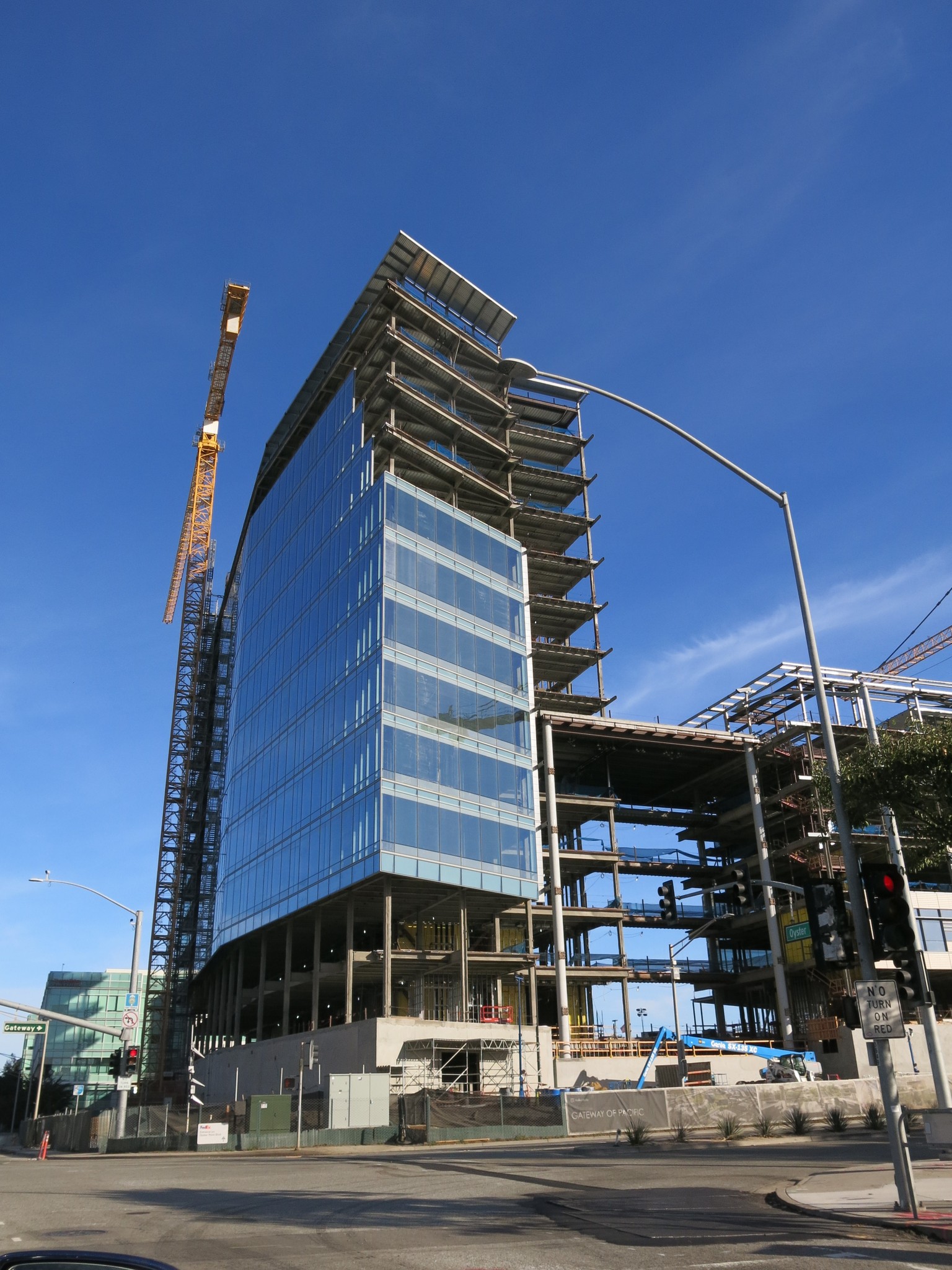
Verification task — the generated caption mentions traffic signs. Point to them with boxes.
[3,1021,49,1034]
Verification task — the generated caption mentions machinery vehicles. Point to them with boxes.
[631,1027,825,1091]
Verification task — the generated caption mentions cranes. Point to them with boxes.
[136,284,249,1100]
[841,586,952,703]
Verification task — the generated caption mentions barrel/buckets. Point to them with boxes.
[536,1087,595,1108]
[500,1088,515,1106]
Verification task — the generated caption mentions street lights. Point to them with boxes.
[496,354,917,1217]
[25,871,143,1137]
[668,913,738,1087]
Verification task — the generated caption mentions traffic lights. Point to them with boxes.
[861,863,919,954]
[302,1040,320,1071]
[108,1045,138,1078]
[804,876,854,971]
[731,866,755,909]
[658,880,679,921]
